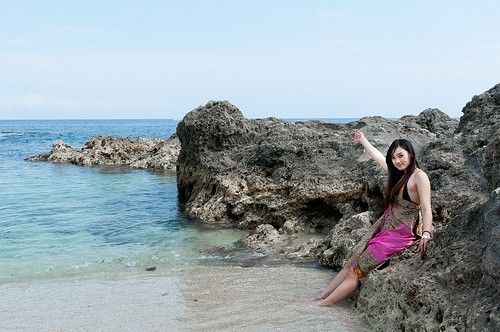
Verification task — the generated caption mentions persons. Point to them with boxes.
[301,129,432,308]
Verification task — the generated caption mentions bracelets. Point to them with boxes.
[421,231,431,237]
